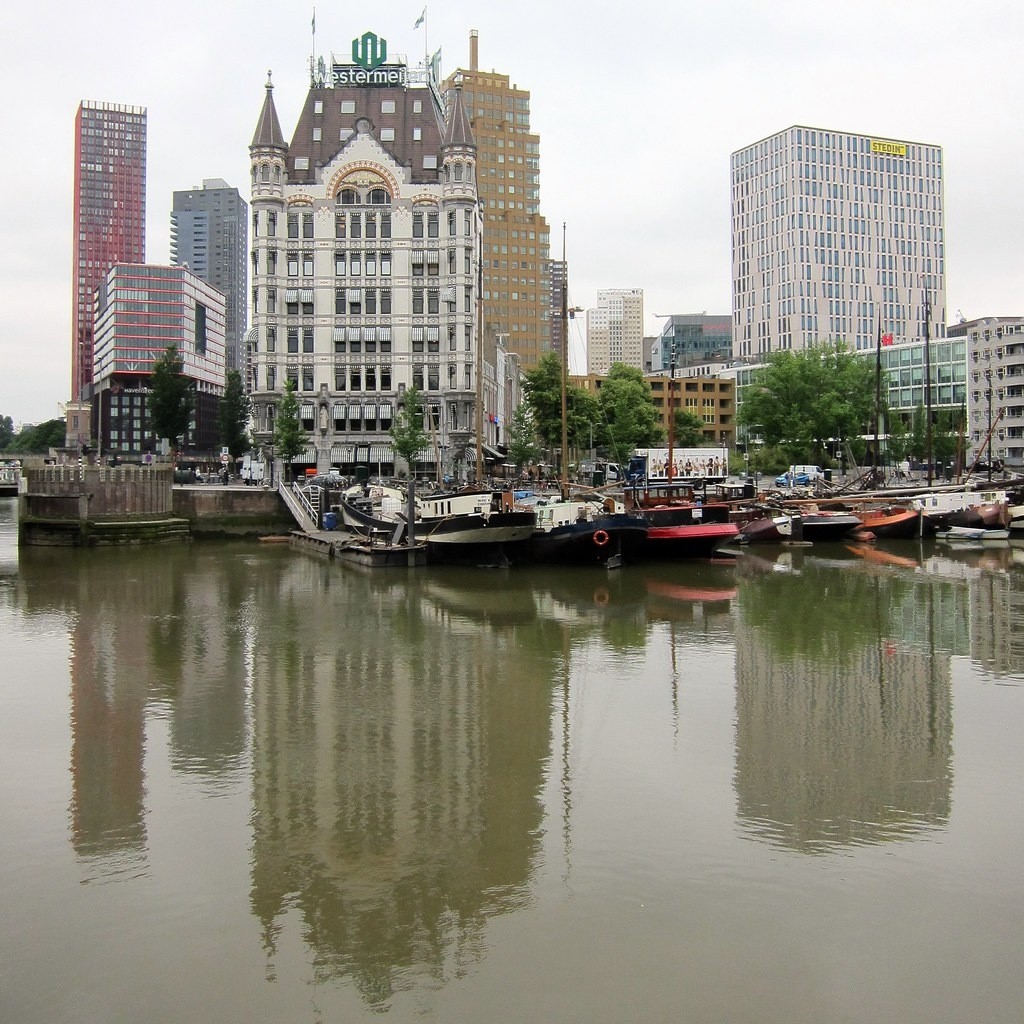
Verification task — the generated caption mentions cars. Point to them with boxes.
[968,460,1004,474]
[774,472,809,487]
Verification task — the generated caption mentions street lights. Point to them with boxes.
[590,421,602,481]
[98,356,102,457]
[442,421,452,476]
[249,439,254,485]
[348,450,351,488]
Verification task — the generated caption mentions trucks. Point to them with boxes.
[626,448,728,490]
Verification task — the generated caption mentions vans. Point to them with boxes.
[790,466,825,481]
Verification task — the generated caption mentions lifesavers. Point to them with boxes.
[593,530,609,545]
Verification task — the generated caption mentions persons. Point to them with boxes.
[672,456,728,476]
[785,471,793,489]
[650,458,669,477]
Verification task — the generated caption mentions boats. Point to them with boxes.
[730,271,1024,543]
[339,231,537,566]
[515,221,738,568]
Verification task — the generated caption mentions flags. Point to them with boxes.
[414,9,424,29]
[311,15,315,35]
